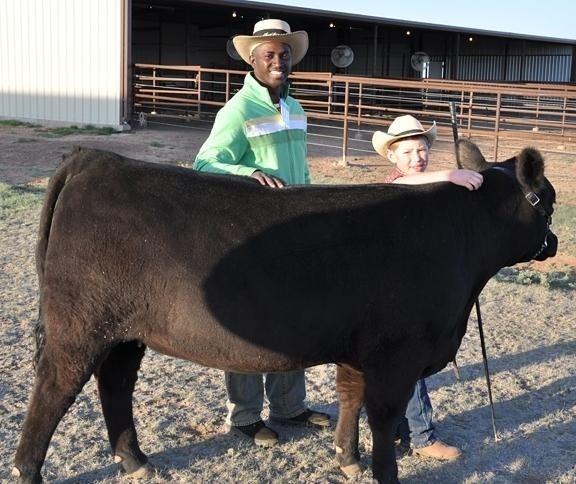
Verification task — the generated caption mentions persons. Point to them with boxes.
[370,115,484,461]
[193,19,330,446]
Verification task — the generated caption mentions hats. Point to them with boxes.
[232,18,309,67]
[372,115,436,158]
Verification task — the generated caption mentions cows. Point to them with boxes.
[11,139,558,484]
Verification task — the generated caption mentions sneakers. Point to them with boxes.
[232,420,278,446]
[412,438,462,460]
[269,408,331,427]
